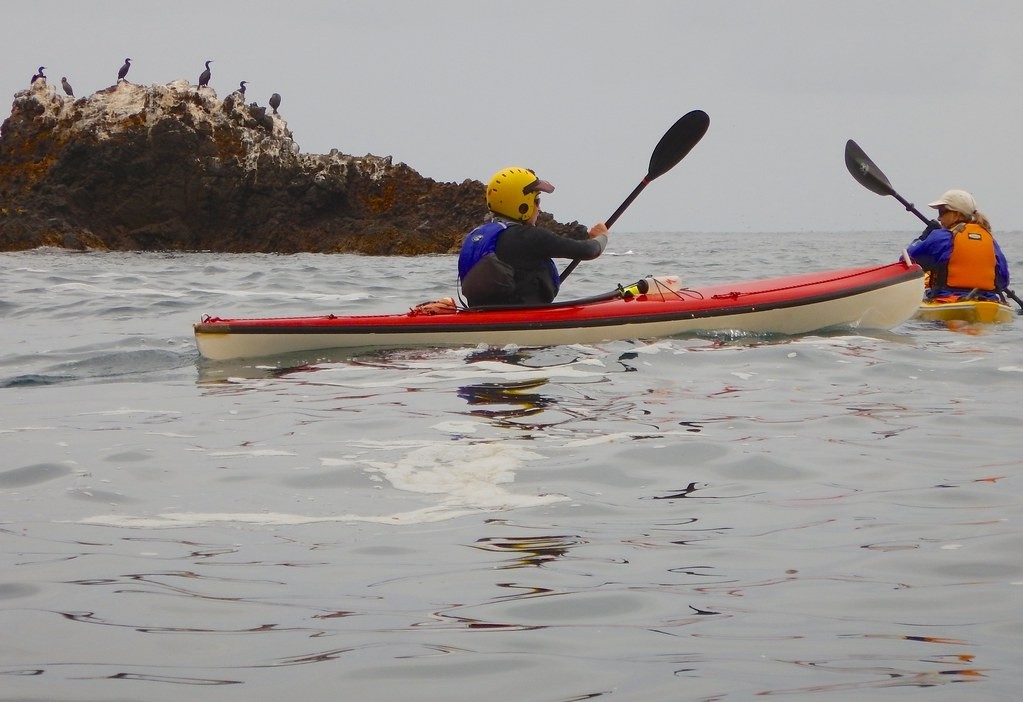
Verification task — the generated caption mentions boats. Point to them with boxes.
[194,264,931,360]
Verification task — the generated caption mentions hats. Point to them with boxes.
[927,189,977,215]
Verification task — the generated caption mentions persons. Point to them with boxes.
[458,167,607,308]
[899,189,1010,307]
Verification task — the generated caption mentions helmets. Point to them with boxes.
[486,167,555,221]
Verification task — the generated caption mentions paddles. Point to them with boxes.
[557,109,710,294]
[844,138,1023,313]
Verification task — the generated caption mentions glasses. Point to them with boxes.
[939,208,952,215]
[533,198,541,207]
